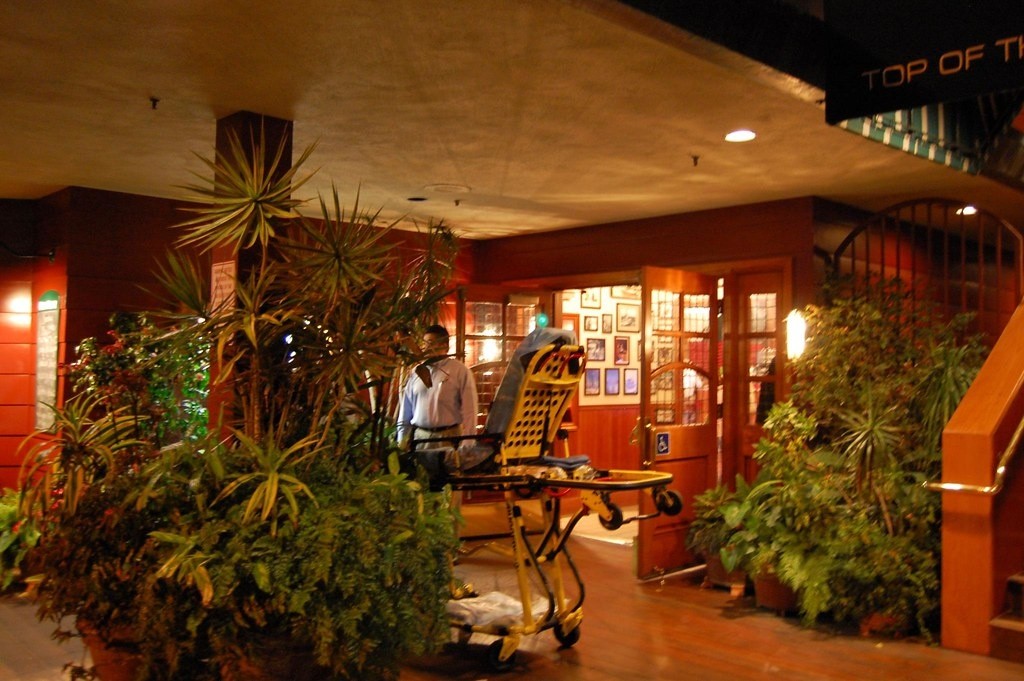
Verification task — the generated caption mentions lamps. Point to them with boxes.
[722,122,759,144]
[785,305,808,361]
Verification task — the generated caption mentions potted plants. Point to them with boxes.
[39,442,454,681]
[692,301,981,641]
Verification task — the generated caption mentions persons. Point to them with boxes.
[756,356,776,424]
[396,325,478,566]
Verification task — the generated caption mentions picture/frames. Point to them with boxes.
[602,314,613,334]
[580,286,602,310]
[584,315,599,332]
[584,368,601,396]
[616,302,642,334]
[610,285,642,301]
[605,368,620,396]
[637,339,642,361]
[614,335,630,366]
[586,337,606,362]
[623,368,639,395]
